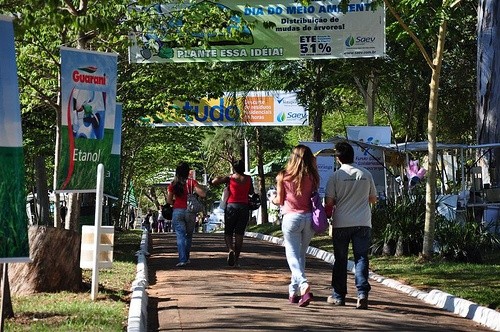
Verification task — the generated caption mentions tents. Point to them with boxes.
[384,142,500,222]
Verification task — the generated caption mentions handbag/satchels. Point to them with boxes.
[185,193,205,212]
[249,193,260,209]
[220,187,229,208]
[311,195,329,233]
[162,203,172,220]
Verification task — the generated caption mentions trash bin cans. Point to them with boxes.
[482,201,500,238]
[74,223,117,270]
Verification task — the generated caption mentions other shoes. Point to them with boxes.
[327,295,344,306]
[289,291,314,307]
[227,249,241,268]
[356,293,369,310]
[176,259,192,266]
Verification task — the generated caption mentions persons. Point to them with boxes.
[267,145,321,307]
[324,141,377,308]
[212,160,254,267]
[195,213,210,233]
[145,205,171,233]
[166,161,206,266]
